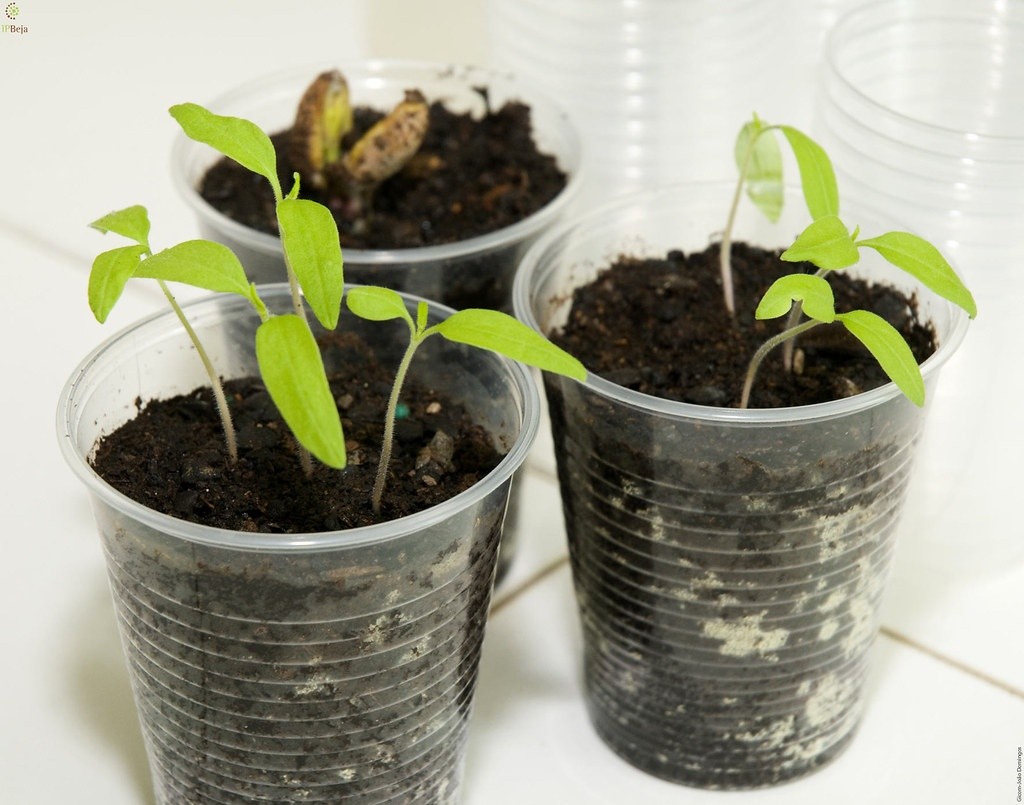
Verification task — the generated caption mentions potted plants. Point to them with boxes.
[55,104,540,805]
[169,64,590,371]
[513,112,979,789]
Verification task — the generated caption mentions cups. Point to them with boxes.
[809,0,1023,286]
